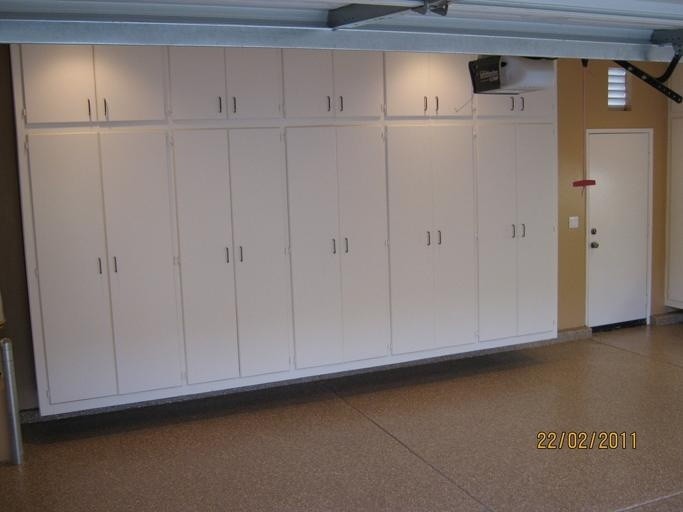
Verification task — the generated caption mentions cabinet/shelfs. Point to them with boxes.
[17,36,166,127]
[283,118,392,371]
[475,58,559,120]
[27,129,178,410]
[386,53,477,123]
[170,46,284,123]
[171,124,295,385]
[473,117,557,346]
[383,120,477,359]
[283,44,387,124]
